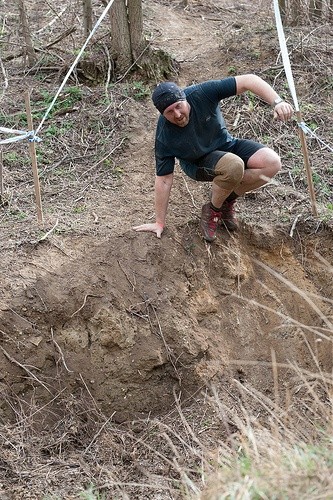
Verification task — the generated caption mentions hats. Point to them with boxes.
[152,82,186,114]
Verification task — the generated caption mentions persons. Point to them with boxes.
[132,72,295,242]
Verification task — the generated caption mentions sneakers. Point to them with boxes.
[200,202,223,242]
[221,200,239,233]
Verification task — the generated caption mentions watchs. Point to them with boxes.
[272,98,284,107]
